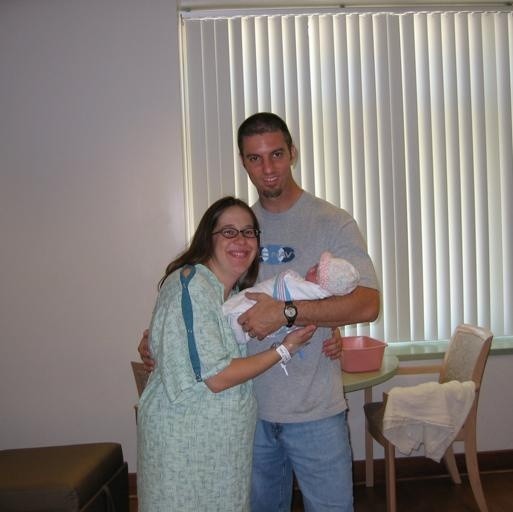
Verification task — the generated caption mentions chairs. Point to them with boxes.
[129,360,156,425]
[362,323,493,511]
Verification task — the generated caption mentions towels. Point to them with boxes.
[382,379,477,464]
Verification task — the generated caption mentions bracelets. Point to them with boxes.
[277,343,293,376]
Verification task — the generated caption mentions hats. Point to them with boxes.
[318,249,359,297]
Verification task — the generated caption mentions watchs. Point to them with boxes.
[284,300,299,327]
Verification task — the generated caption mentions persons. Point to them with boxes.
[138,112,383,512]
[135,197,320,512]
[224,250,360,349]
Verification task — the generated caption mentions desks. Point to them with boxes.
[336,349,399,489]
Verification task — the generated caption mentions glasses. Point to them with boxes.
[210,226,261,239]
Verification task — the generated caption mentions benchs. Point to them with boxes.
[0,441,130,512]
